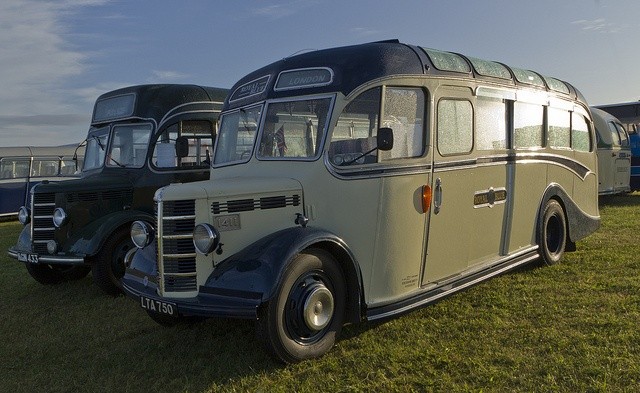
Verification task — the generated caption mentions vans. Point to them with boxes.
[1,147,89,225]
[9,84,234,298]
[589,107,631,196]
[124,38,601,365]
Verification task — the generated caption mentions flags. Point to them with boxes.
[274,126,287,156]
